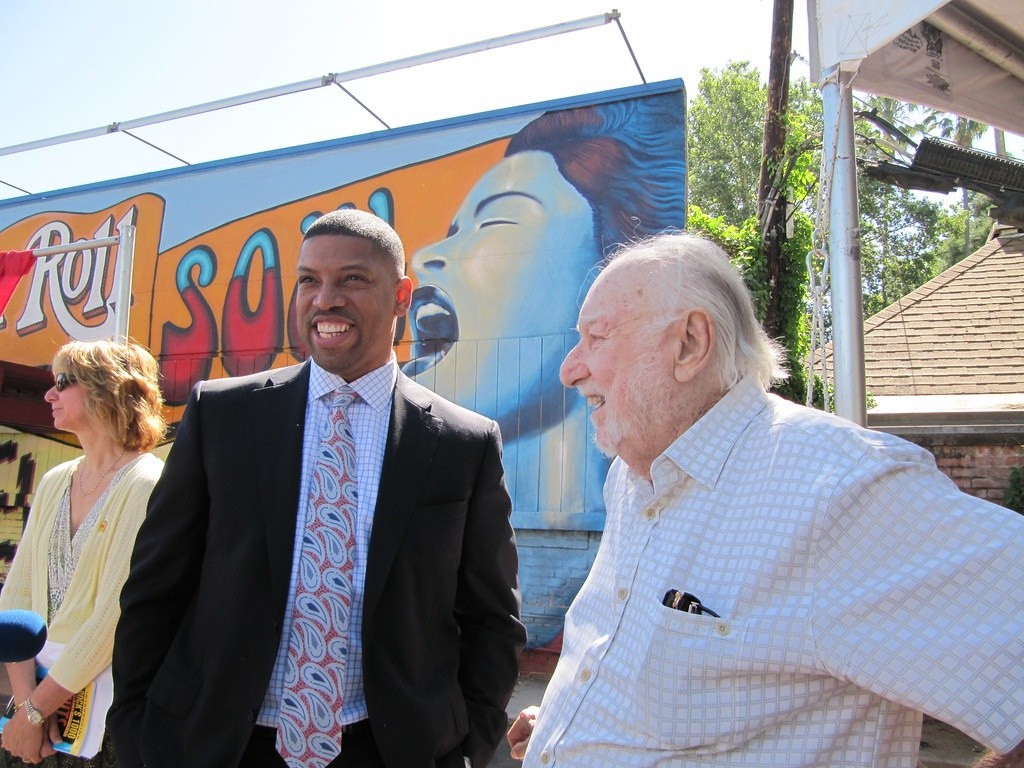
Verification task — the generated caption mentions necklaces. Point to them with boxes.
[79,450,123,495]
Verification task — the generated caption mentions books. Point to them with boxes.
[0,663,97,756]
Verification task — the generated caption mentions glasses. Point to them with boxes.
[55,372,85,392]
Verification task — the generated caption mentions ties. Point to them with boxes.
[276,385,358,768]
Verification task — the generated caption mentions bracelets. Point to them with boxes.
[13,701,25,713]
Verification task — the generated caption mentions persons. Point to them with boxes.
[0,339,166,767]
[102,209,528,768]
[507,232,1024,768]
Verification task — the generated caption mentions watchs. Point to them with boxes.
[25,697,44,726]
